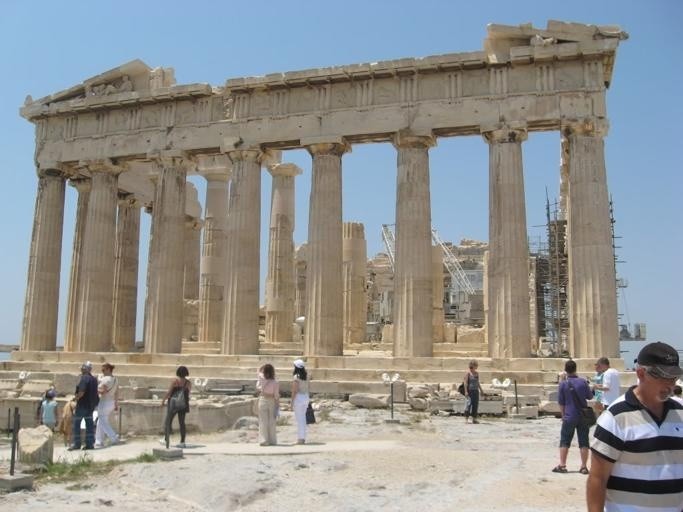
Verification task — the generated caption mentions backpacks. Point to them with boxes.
[168,379,187,411]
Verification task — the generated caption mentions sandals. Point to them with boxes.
[552,465,568,472]
[580,467,589,474]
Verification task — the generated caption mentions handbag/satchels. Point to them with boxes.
[579,407,596,425]
[306,404,315,424]
[457,381,468,397]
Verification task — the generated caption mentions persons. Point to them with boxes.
[463,360,483,424]
[587,342,683,512]
[290,359,310,445]
[35,360,120,451]
[160,367,191,449]
[669,385,683,406]
[552,361,595,473]
[594,357,621,410]
[587,363,604,413]
[256,364,280,446]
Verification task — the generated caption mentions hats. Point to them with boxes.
[293,359,305,369]
[81,361,93,370]
[47,390,56,397]
[634,341,683,376]
